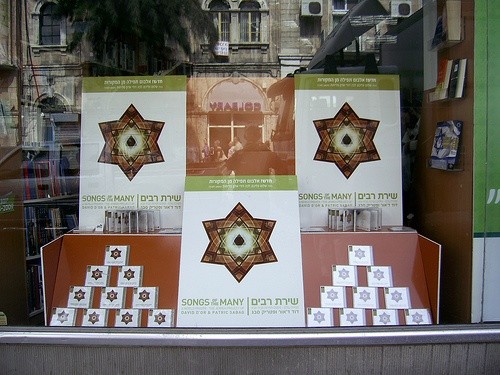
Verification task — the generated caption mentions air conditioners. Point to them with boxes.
[388,0,414,19]
[299,0,323,19]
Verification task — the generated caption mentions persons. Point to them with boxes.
[209,137,243,175]
[226,124,289,175]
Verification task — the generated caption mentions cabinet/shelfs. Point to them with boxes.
[0,145,81,324]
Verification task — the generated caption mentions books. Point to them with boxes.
[431,120,464,168]
[438,0,463,49]
[429,58,469,100]
[23,158,80,311]
[177,175,306,328]
[186,77,296,175]
[295,73,403,231]
[78,75,185,235]
